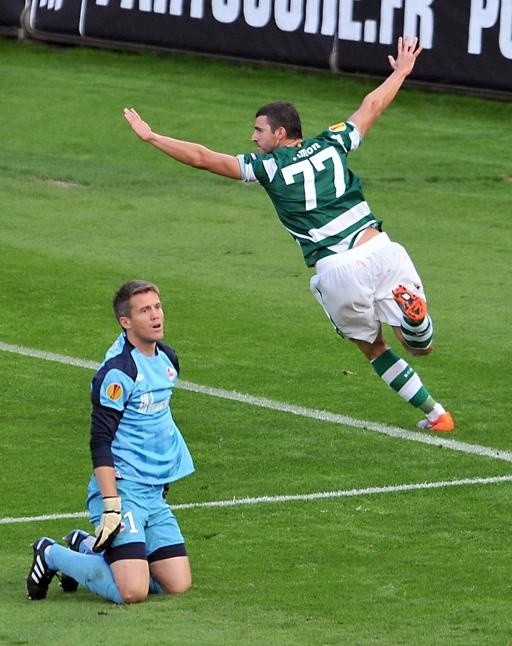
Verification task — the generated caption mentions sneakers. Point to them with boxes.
[24,537,55,600]
[390,284,427,324]
[56,528,90,593]
[415,410,455,432]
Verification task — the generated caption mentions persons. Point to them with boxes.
[27,280,196,606]
[123,33,455,431]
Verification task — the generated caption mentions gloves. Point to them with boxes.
[92,495,122,553]
